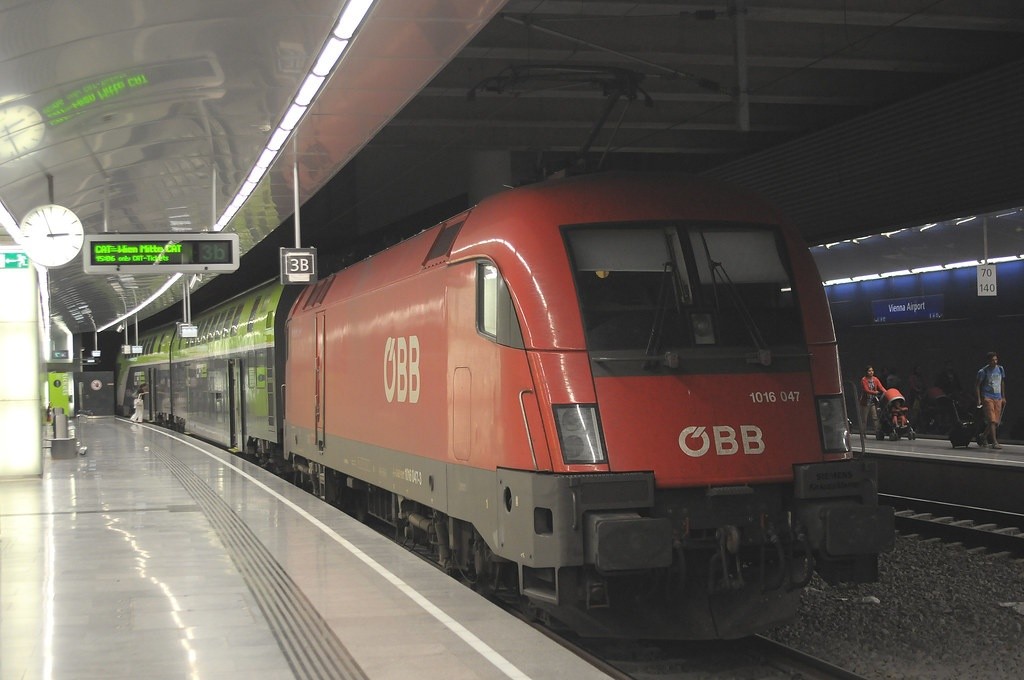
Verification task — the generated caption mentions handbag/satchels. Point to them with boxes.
[135,394,143,406]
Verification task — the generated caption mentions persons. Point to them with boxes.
[889,401,908,428]
[860,366,887,439]
[975,352,1006,449]
[130,383,149,423]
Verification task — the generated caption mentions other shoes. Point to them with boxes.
[991,442,1002,449]
[977,433,989,448]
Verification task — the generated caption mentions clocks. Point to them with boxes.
[18,204,85,269]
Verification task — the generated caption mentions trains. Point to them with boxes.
[116,164,898,643]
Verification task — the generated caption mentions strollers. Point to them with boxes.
[870,388,917,440]
